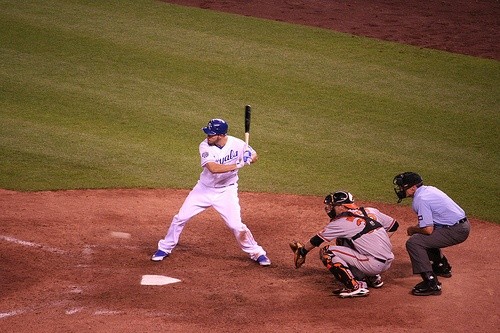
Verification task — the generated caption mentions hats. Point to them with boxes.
[401,172,422,190]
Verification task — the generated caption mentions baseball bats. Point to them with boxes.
[244,105,252,163]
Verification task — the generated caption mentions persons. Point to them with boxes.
[393,171,471,296]
[151,119,272,266]
[289,190,399,297]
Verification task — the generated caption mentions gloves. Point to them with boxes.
[236,150,252,168]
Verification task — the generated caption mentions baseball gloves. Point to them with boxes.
[289,240,306,269]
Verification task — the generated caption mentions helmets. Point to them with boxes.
[323,193,334,203]
[332,192,355,203]
[202,119,228,135]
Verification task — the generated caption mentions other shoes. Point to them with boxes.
[151,250,168,262]
[337,281,370,297]
[360,274,383,288]
[432,263,452,278]
[412,280,442,296]
[258,255,271,266]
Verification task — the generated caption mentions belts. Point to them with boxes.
[458,216,467,223]
[373,257,386,264]
